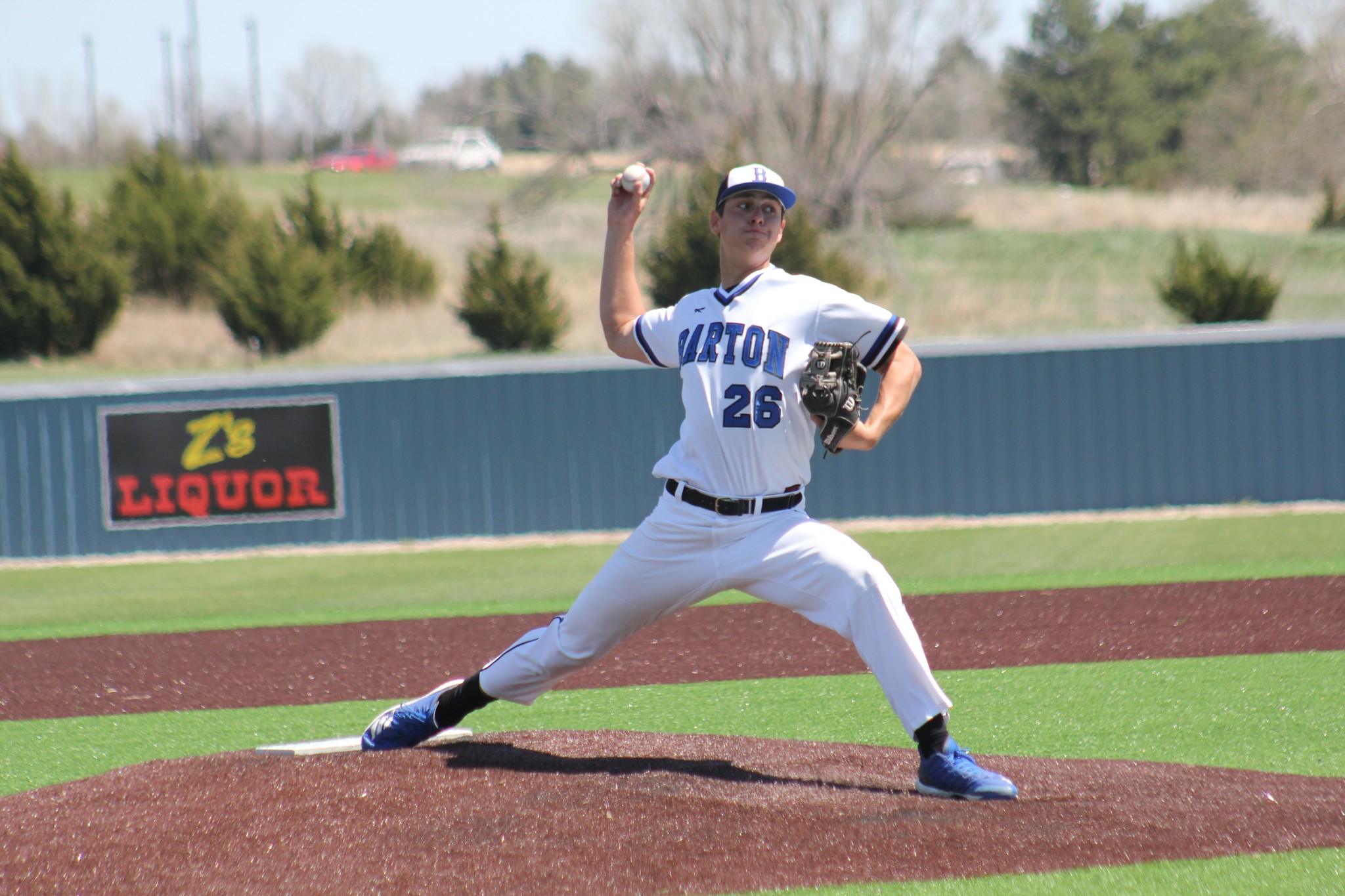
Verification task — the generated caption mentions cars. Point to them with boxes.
[311,143,395,173]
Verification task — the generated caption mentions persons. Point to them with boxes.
[362,162,1022,802]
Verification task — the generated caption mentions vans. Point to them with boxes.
[401,127,501,173]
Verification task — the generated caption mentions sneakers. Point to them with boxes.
[361,680,465,750]
[915,737,1019,800]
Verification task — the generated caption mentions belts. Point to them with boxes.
[666,478,802,516]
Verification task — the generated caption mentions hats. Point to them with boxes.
[716,163,796,209]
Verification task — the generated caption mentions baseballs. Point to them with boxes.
[621,164,651,195]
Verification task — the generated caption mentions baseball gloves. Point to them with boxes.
[798,328,871,460]
